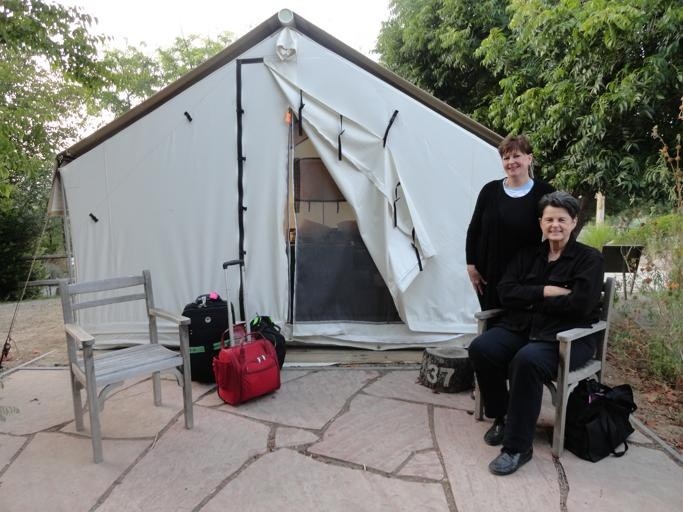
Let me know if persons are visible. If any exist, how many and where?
[465,137,558,327]
[469,192,603,475]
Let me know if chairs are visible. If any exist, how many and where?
[475,276,619,461]
[58,269,196,462]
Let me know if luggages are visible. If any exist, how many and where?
[212,259,282,406]
[179,295,235,385]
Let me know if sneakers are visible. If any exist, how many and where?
[489,446,533,475]
[484,418,503,446]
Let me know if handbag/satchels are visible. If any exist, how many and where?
[563,376,638,463]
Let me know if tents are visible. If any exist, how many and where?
[57,9,505,350]
